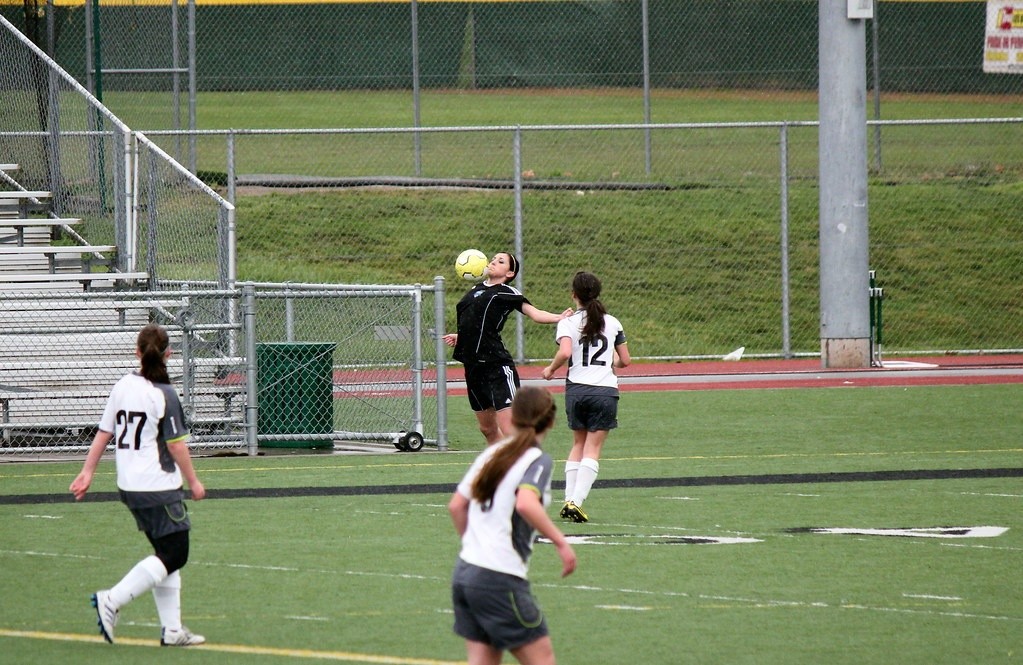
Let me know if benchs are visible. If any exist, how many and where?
[0,163,247,441]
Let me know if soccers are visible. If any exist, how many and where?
[454,248,490,282]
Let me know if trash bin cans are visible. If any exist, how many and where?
[254,340,339,449]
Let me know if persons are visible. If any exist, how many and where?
[542,272,631,523]
[441,252,574,446]
[70,324,205,646]
[450,386,576,665]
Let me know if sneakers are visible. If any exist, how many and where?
[89,591,115,644]
[567,504,588,523]
[560,502,572,518]
[161,627,206,647]
